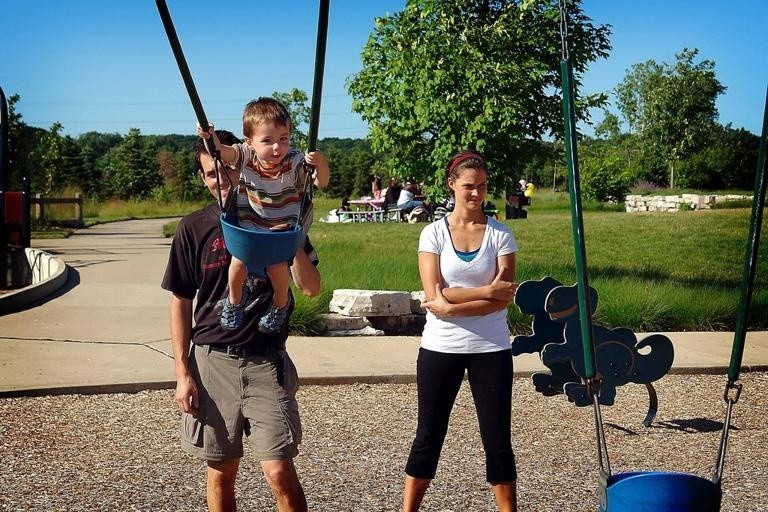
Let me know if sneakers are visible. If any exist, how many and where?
[220,284,251,334]
[258,290,294,336]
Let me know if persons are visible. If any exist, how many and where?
[196,97,330,335]
[160,131,321,512]
[339,175,426,221]
[403,150,519,512]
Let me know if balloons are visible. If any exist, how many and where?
[519,180,534,198]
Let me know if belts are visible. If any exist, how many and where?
[197,342,286,356]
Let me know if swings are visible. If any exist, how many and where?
[560,1,768,510]
[156,0,330,275]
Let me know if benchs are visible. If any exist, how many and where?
[337,193,500,224]
